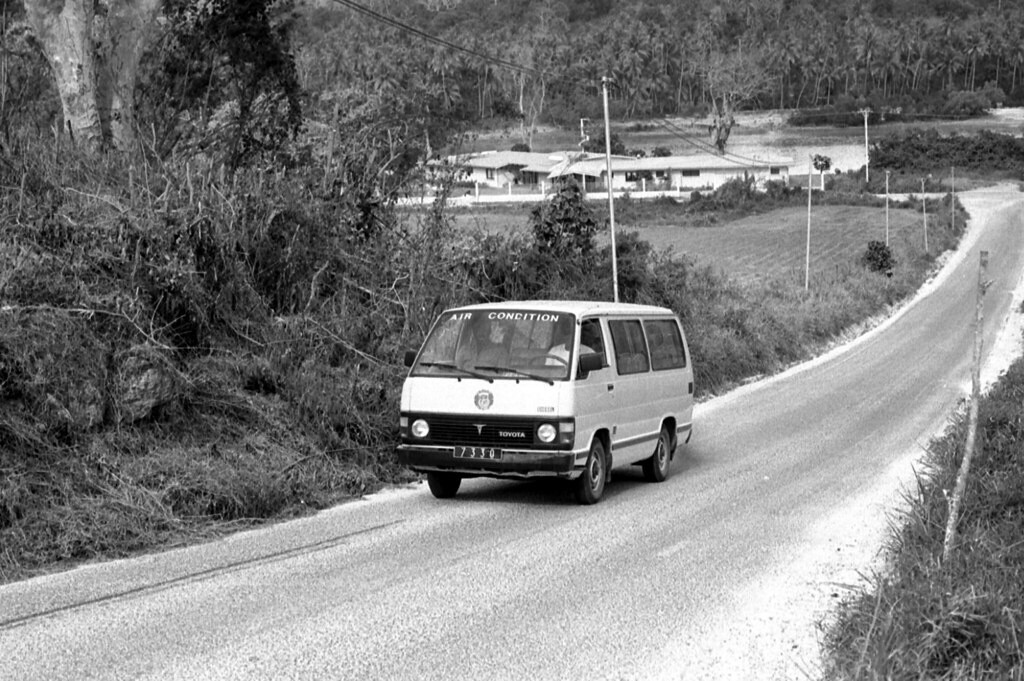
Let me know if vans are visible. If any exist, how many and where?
[396,297,696,505]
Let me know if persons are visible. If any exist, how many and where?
[455,318,507,369]
[545,319,596,366]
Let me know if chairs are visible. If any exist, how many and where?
[476,347,509,373]
[618,354,647,373]
[651,345,682,371]
